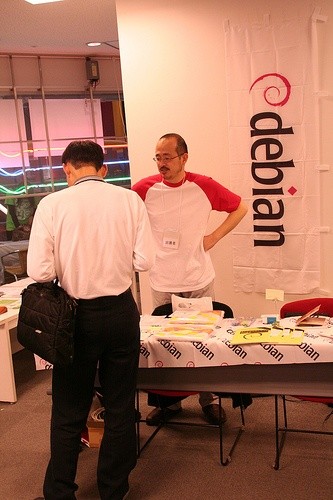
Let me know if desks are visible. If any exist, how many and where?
[35,314,333,398]
[0,277,61,404]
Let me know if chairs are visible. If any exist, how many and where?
[275,297,333,469]
[136,301,246,466]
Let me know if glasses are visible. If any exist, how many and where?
[153,153,183,163]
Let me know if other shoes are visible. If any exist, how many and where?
[109,483,130,500]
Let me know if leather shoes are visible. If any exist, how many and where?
[146,407,182,425]
[202,404,226,425]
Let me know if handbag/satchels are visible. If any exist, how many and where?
[16,281,77,364]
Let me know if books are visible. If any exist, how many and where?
[230,305,326,346]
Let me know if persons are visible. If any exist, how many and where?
[127,133,249,426]
[26,141,155,500]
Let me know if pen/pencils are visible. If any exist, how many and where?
[240,330,268,333]
